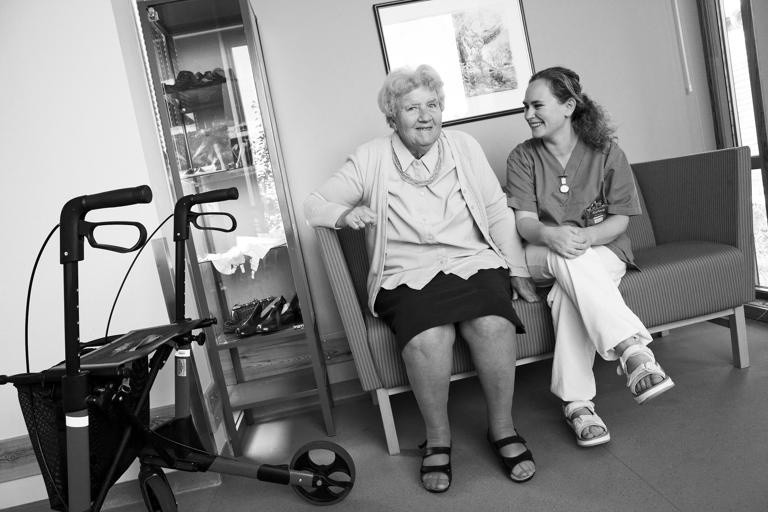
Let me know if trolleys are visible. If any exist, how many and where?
[0,184,356,512]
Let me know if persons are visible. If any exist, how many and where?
[504,63,676,451]
[300,62,541,496]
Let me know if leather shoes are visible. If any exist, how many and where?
[235,293,302,336]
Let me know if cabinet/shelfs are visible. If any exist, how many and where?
[147,1,338,457]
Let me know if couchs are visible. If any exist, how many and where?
[312,142,756,461]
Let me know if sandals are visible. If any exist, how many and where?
[486,424,536,483]
[560,401,611,446]
[417,437,451,492]
[616,344,675,407]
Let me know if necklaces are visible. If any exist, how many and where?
[387,133,446,189]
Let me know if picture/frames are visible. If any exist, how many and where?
[373,0,538,133]
